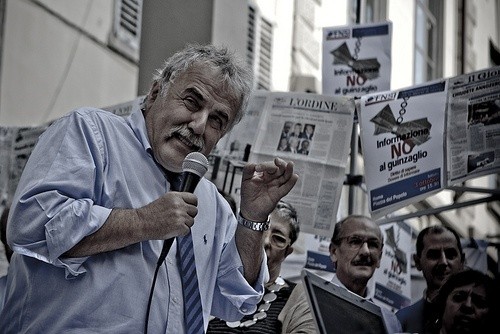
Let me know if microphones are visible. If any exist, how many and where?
[157,152,208,266]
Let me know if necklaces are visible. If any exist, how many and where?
[220,274,284,329]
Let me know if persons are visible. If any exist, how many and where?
[277,122,315,156]
[0,40,299,334]
[208,199,500,334]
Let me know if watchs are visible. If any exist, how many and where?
[236,209,271,232]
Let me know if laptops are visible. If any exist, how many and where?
[301,269,389,334]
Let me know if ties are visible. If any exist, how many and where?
[167,171,205,334]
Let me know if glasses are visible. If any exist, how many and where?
[264,232,290,251]
[339,235,383,250]
